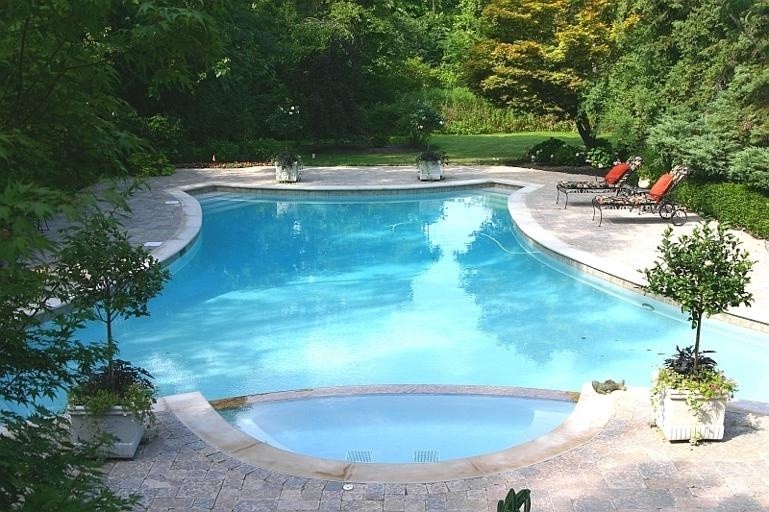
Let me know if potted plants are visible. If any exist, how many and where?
[46,200,174,462]
[644,211,761,445]
[413,148,448,184]
[273,151,304,185]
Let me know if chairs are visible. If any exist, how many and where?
[553,153,690,226]
